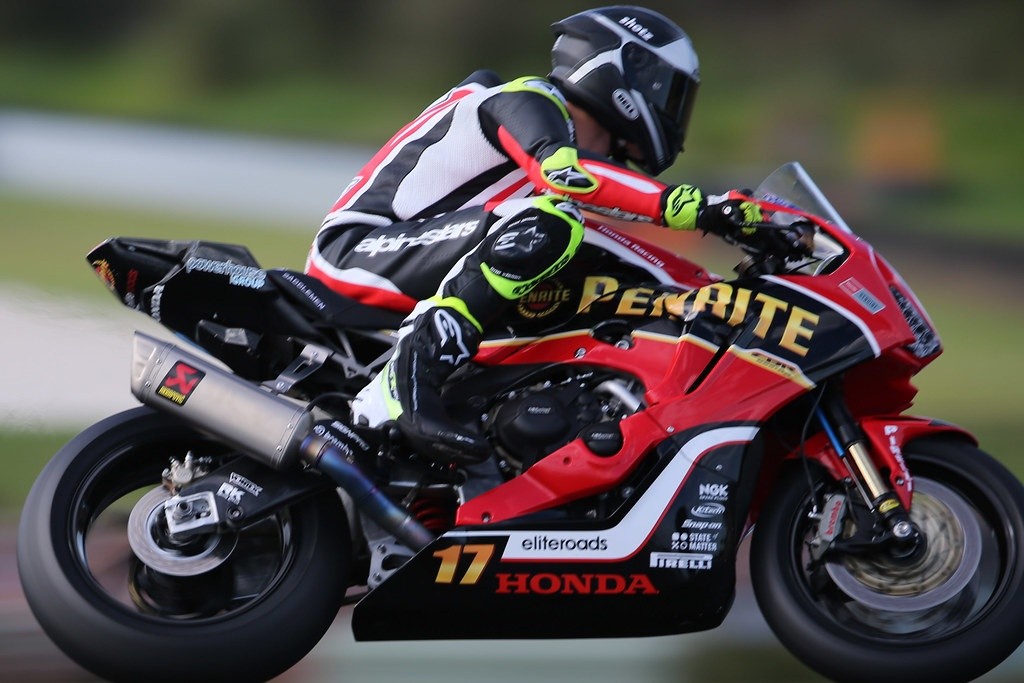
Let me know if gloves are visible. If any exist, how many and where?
[703,189,817,264]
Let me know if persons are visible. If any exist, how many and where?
[303,5,814,466]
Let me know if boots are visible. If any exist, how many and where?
[350,296,494,464]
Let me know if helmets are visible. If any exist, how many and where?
[547,5,700,176]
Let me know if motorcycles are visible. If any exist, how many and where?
[13,160,1024,683]
